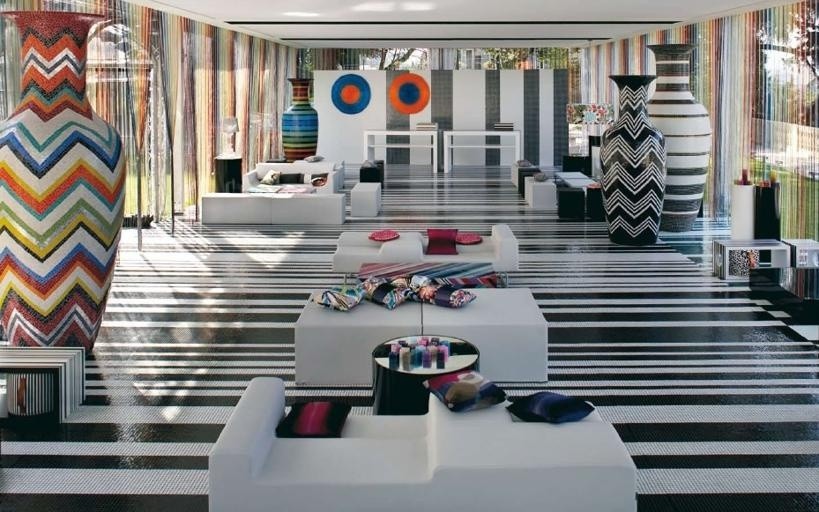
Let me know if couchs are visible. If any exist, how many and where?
[243,162,337,194]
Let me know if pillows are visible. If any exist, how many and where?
[260,170,328,187]
[427,229,458,256]
[422,372,504,412]
[274,400,350,437]
[310,275,477,314]
[507,391,594,426]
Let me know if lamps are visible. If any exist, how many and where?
[222,117,239,157]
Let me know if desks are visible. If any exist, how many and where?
[443,130,522,175]
[363,129,438,175]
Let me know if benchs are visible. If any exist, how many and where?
[360,161,384,189]
[295,286,548,386]
[332,224,519,288]
[511,165,558,212]
[0,347,85,423]
[202,192,346,226]
[208,377,636,512]
[350,183,381,217]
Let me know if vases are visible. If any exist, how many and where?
[282,79,318,162]
[0,11,126,356]
[600,75,668,247]
[646,44,712,232]
